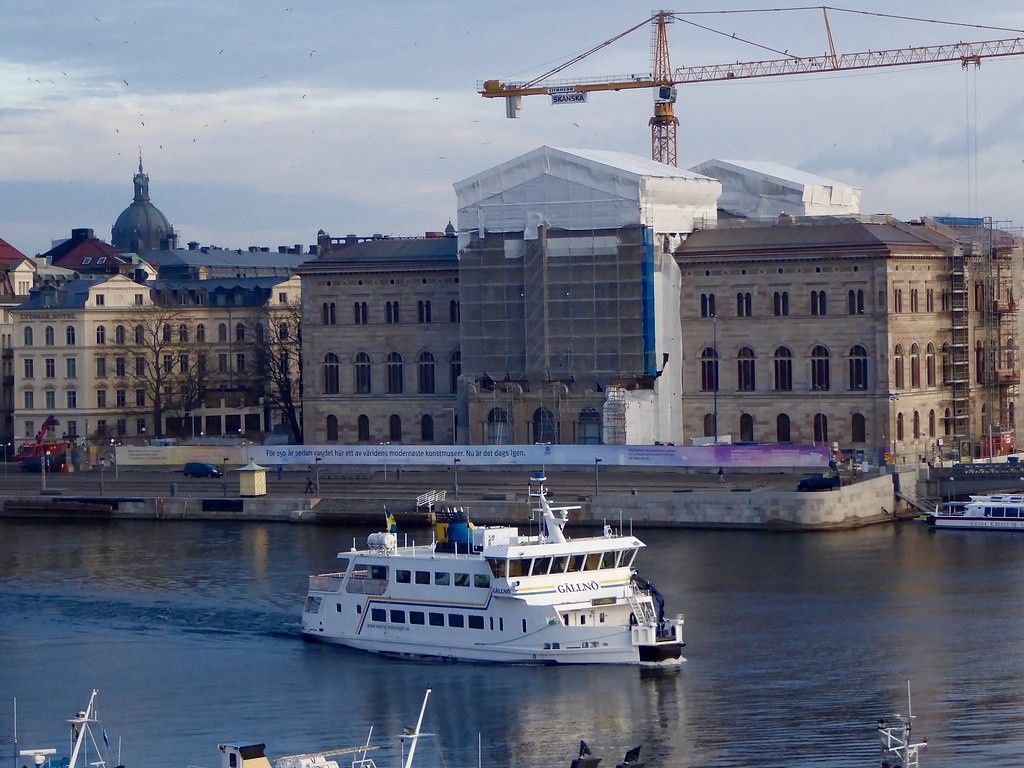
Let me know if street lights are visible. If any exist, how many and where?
[223,456,229,498]
[442,408,456,445]
[315,455,322,496]
[0,442,11,476]
[710,313,719,443]
[535,442,553,478]
[595,456,602,497]
[452,456,461,499]
[99,457,105,497]
[814,384,826,447]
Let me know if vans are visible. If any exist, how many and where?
[183,461,225,479]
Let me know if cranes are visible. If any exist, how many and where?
[473,7,1024,168]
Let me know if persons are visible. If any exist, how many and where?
[304,477,314,494]
[277,464,283,480]
[996,448,1000,456]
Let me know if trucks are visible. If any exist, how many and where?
[17,414,70,473]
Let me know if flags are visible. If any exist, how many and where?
[383,505,398,534]
[72,722,79,742]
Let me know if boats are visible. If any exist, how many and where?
[912,494,1024,529]
[300,471,687,665]
